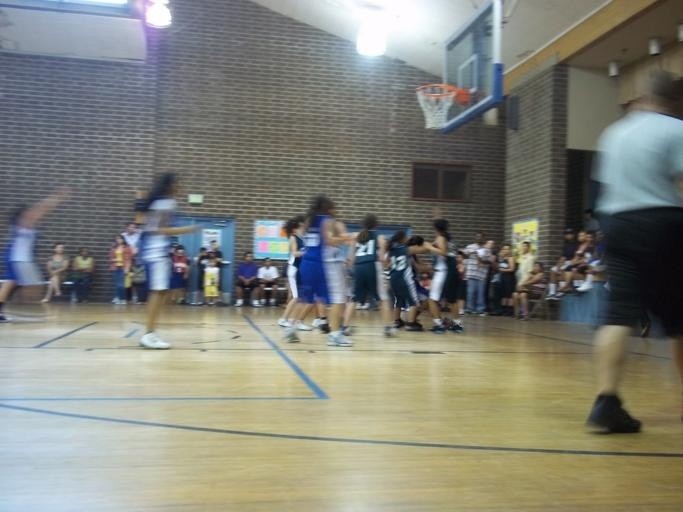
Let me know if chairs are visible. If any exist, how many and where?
[517,278,553,323]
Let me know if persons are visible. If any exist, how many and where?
[584,69,681,431]
[0,171,652,351]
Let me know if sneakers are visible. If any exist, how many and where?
[384,318,463,336]
[234,299,276,307]
[586,396,640,434]
[545,285,594,301]
[285,334,301,342]
[327,336,352,347]
[458,310,529,321]
[113,298,147,305]
[355,301,381,311]
[140,333,170,349]
[278,318,353,336]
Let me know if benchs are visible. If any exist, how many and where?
[11,278,86,303]
[243,283,288,304]
